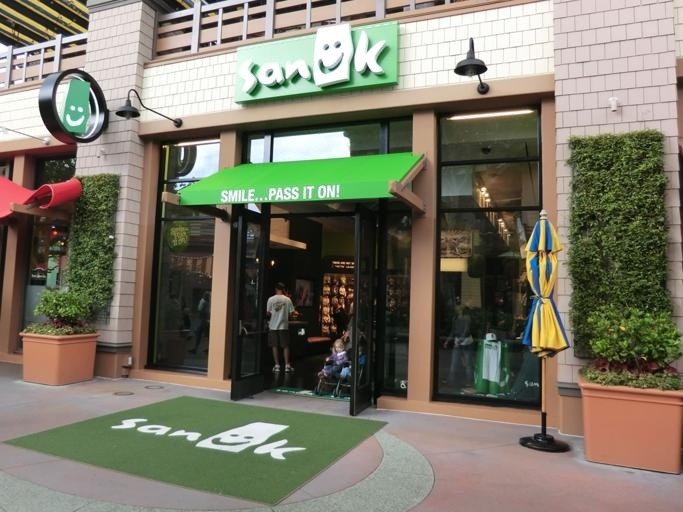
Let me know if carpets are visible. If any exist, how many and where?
[1,393,390,506]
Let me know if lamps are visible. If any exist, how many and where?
[453,36,491,99]
[114,87,184,131]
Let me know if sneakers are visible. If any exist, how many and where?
[272,364,280,372]
[285,365,295,372]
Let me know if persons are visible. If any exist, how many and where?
[317,338,348,379]
[340,317,352,340]
[265,282,298,372]
[329,303,348,337]
[186,273,209,356]
[440,304,474,386]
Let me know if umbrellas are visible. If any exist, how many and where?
[521,209,571,434]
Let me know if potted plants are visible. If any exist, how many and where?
[19,287,102,386]
[156,306,192,365]
[574,302,683,476]
